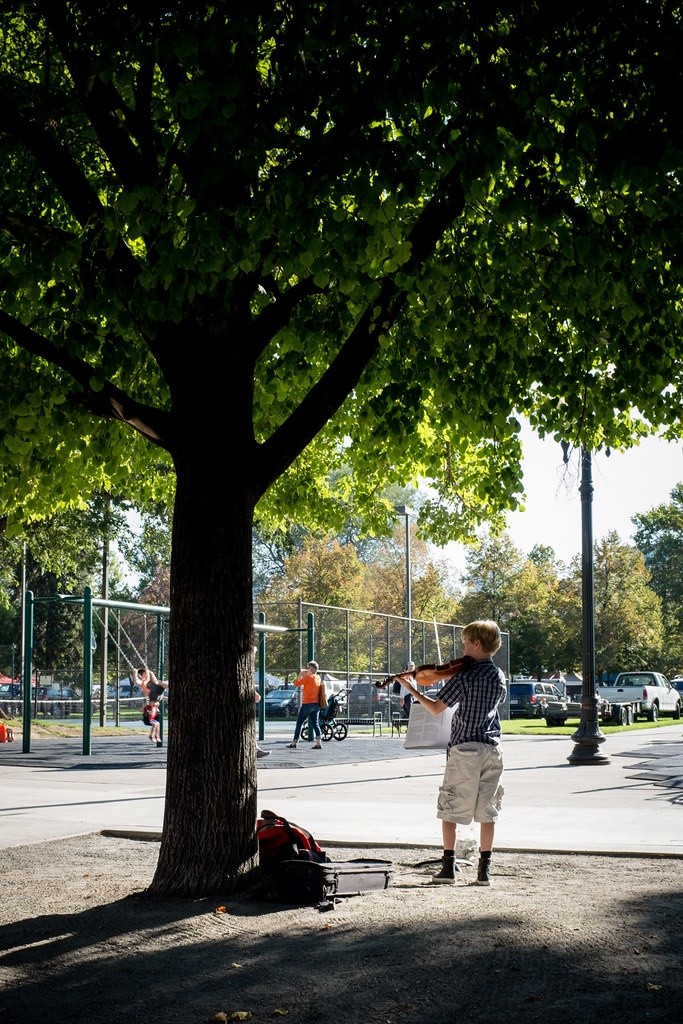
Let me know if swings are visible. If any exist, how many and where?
[92,607,164,701]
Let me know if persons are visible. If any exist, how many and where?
[134,669,169,743]
[286,659,321,750]
[540,697,548,715]
[254,690,269,758]
[399,660,417,716]
[395,620,507,886]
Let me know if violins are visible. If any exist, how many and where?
[375,655,476,691]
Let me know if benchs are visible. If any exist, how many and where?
[333,711,383,738]
[390,712,408,737]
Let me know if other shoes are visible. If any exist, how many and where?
[286,744,296,748]
[257,749,269,758]
[311,744,322,749]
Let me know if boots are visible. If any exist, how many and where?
[476,858,491,886]
[432,856,455,883]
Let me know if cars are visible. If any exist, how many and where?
[256,683,298,717]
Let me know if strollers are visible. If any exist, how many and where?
[300,688,352,742]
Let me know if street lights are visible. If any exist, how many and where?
[391,506,413,661]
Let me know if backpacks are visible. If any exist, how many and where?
[256,809,329,873]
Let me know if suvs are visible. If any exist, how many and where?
[347,681,441,718]
[669,675,683,717]
[507,682,569,719]
[0,676,168,719]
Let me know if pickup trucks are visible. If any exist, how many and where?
[596,671,682,722]
